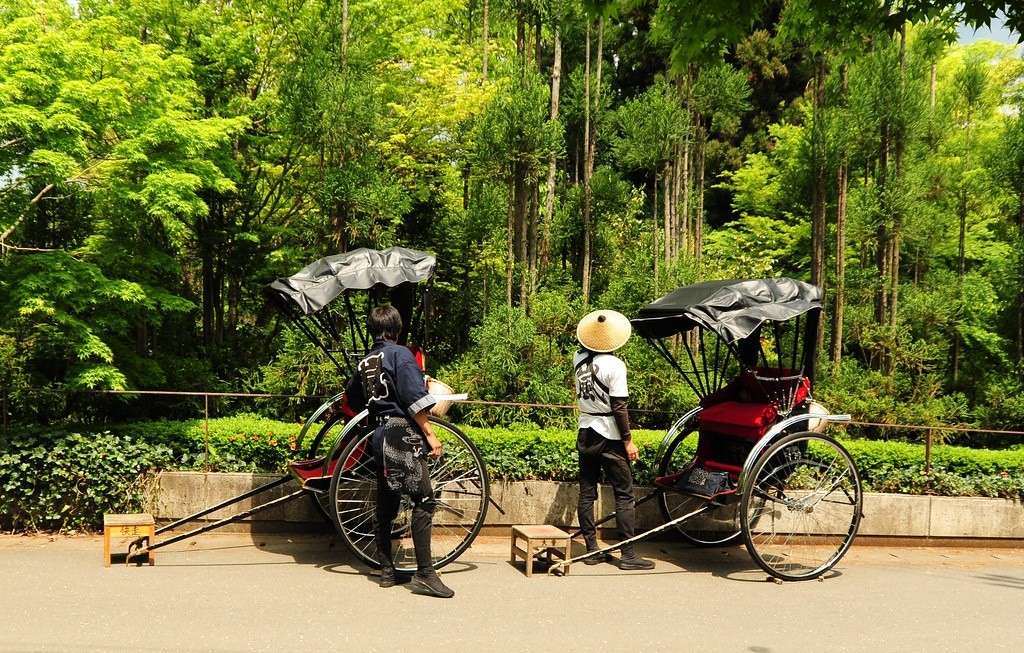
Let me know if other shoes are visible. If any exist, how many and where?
[412,571,454,598]
[380,567,412,587]
[585,550,613,565]
[620,557,656,569]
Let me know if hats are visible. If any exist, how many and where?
[576,310,631,352]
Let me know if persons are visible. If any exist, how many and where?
[572,310,656,569]
[345,304,454,599]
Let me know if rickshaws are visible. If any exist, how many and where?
[544,276,865,582]
[125,245,493,581]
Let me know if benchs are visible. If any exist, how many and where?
[284,344,425,496]
[693,364,811,443]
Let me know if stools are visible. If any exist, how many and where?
[102,513,156,567]
[510,524,572,579]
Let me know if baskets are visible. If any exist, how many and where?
[423,377,456,416]
